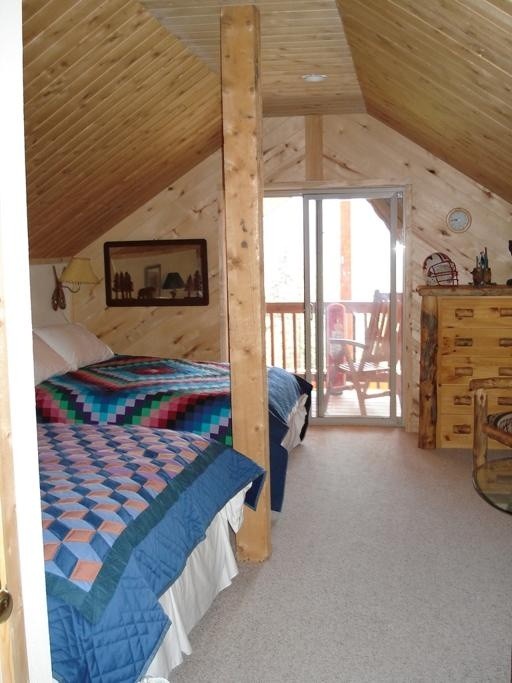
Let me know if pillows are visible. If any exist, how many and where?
[33,320,112,386]
[34,334,68,386]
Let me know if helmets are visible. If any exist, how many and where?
[424,253,458,286]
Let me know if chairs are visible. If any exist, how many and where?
[469,377,512,514]
[322,289,404,417]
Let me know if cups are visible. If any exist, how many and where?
[472,267,491,286]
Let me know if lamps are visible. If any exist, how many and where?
[51,257,97,311]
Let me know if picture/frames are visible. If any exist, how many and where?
[104,239,209,306]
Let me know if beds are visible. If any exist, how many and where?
[36,354,313,510]
[36,423,267,683]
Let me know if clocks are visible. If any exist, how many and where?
[446,208,472,234]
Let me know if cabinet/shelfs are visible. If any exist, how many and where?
[418,285,512,449]
